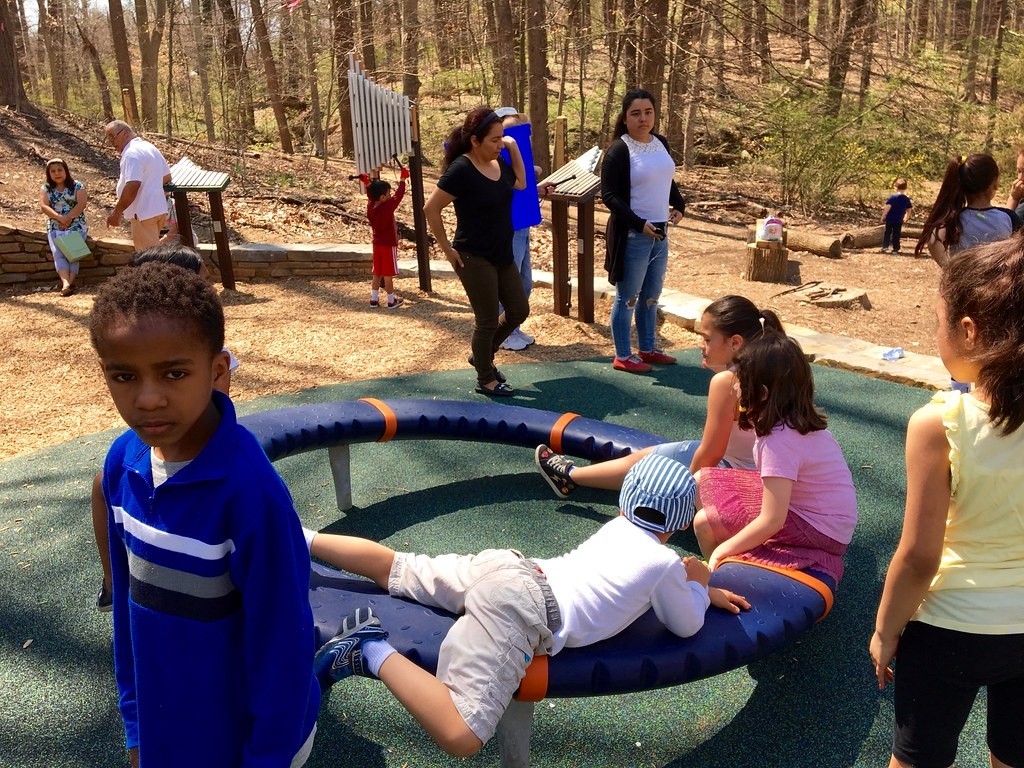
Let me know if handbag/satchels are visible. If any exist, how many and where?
[53,227,91,263]
[756,214,774,243]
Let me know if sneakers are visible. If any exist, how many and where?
[499,335,529,350]
[370,297,379,307]
[510,327,535,344]
[314,606,389,692]
[388,298,403,309]
[613,356,652,371]
[536,445,576,499]
[637,349,677,363]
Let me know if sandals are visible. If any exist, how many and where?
[476,381,515,395]
[469,355,505,381]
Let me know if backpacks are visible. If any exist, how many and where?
[761,215,782,241]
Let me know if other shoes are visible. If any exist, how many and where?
[894,250,898,254]
[62,286,70,295]
[881,248,886,253]
[95,578,113,611]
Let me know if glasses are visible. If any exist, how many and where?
[110,129,124,141]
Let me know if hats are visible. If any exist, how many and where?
[618,453,697,534]
[493,107,528,123]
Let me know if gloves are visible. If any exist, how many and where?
[401,168,410,178]
[359,173,369,185]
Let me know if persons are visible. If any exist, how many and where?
[492,108,557,350]
[90,261,321,768]
[423,105,530,396]
[105,120,199,253]
[300,452,752,756]
[40,157,88,296]
[600,88,685,373]
[359,166,410,310]
[535,294,786,499]
[880,179,913,254]
[91,239,239,613]
[869,227,1024,768]
[692,328,858,573]
[916,149,1024,394]
